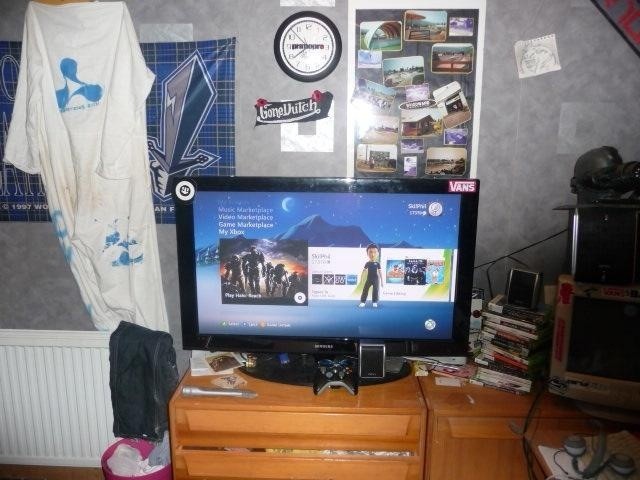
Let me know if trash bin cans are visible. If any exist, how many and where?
[101,437,172,480]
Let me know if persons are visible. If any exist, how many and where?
[358,243,383,308]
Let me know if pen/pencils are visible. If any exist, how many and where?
[466,393,474,404]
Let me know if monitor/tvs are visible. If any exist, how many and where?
[172,176,479,386]
[548,275,640,423]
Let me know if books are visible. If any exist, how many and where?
[469,295,554,396]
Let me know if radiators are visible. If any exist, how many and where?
[0,327,123,468]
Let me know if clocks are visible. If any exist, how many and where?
[275,9,342,82]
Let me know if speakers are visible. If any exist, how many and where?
[568,210,639,283]
[505,267,543,311]
[358,339,385,379]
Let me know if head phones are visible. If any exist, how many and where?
[562,434,635,477]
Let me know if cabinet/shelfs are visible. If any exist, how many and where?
[413,360,590,480]
[167,355,428,479]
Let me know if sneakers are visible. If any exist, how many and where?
[359,303,365,307]
[373,303,377,307]
